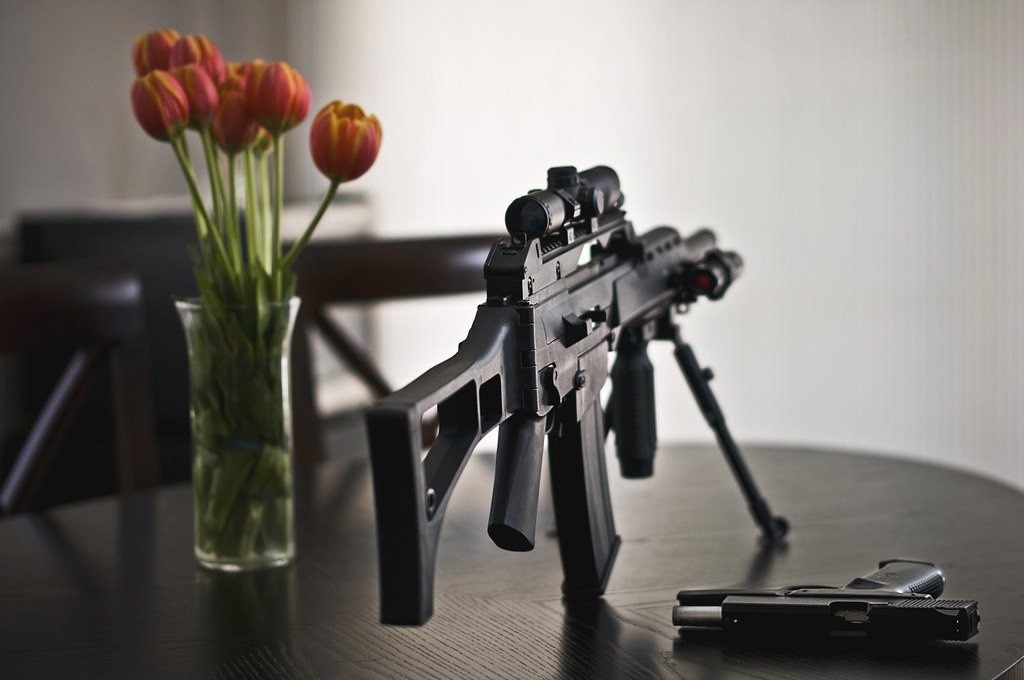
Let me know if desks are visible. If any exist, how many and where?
[0,437,1024,680]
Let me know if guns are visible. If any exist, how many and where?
[674,560,979,644]
[367,163,790,626]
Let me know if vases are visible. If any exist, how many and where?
[170,298,305,571]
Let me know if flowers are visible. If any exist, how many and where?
[128,25,382,561]
